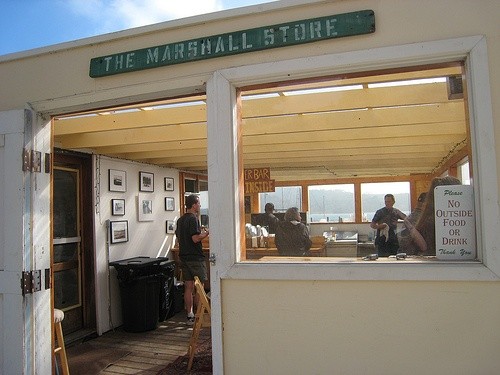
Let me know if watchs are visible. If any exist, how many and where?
[205,230,209,234]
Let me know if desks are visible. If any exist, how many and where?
[170,236,326,288]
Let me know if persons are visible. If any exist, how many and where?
[402,177,463,254]
[370,194,407,256]
[175,194,209,326]
[274,207,312,256]
[254,203,280,234]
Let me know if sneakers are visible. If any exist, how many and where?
[187,313,195,326]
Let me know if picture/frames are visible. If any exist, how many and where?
[140,171,154,191]
[165,177,175,190]
[112,199,125,215]
[109,169,127,192]
[165,197,175,211]
[139,193,156,221]
[167,220,176,233]
[111,220,128,244]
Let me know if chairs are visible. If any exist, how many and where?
[186,276,211,372]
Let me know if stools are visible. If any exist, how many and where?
[54,308,69,375]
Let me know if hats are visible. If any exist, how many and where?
[417,191,430,202]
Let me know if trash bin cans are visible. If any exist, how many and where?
[148,258,177,322]
[108,255,168,331]
